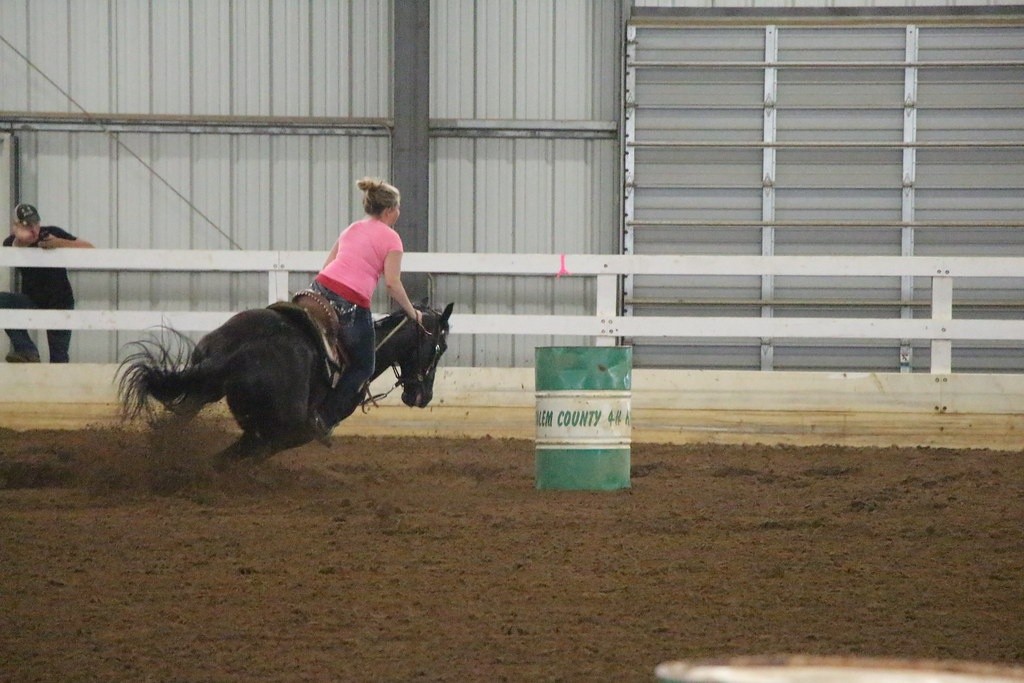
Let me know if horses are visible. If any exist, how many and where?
[108,287,456,484]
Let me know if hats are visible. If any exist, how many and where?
[13,204,40,226]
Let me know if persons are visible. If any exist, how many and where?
[0,204,95,363]
[309,176,423,448]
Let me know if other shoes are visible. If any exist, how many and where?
[306,409,332,448]
[6,351,41,363]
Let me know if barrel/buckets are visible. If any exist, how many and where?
[532,343,633,489]
